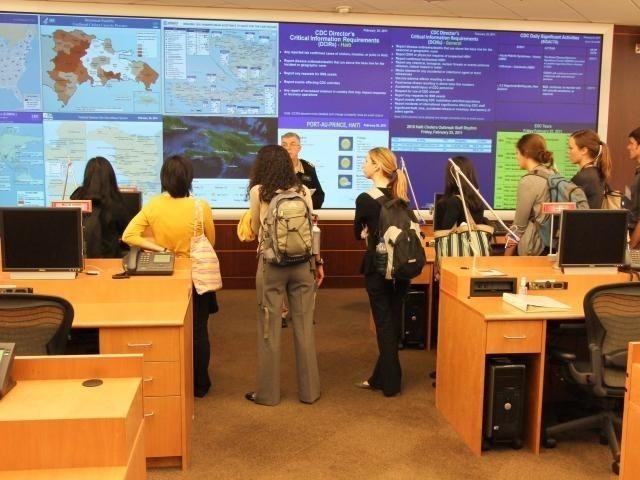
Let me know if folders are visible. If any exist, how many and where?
[501,291,573,314]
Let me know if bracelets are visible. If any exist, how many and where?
[160,247,170,253]
[121,153,223,398]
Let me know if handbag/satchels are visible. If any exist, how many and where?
[435,192,495,274]
[602,178,622,210]
[190,200,227,295]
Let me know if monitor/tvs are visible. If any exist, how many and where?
[555,208,629,275]
[0,206,85,280]
[121,191,141,222]
[432,191,448,232]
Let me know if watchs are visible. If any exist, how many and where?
[313,259,325,267]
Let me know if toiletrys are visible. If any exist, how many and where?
[519,278,527,294]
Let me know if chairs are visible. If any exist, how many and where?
[0,291,75,355]
[545,281,640,474]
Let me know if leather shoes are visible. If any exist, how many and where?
[246,391,266,403]
[353,380,374,389]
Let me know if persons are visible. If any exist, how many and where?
[568,129,614,210]
[269,132,325,328]
[427,155,495,386]
[69,156,137,259]
[352,147,411,397]
[503,134,566,256]
[236,145,325,406]
[622,126,640,252]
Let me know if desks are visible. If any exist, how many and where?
[0,256,195,470]
[367,224,439,351]
[0,352,147,480]
[433,256,640,459]
[617,341,640,480]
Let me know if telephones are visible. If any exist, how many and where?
[111,243,176,280]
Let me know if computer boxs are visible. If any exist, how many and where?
[402,289,428,345]
[483,359,528,443]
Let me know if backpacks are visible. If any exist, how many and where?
[256,183,316,266]
[521,165,591,254]
[361,188,427,284]
